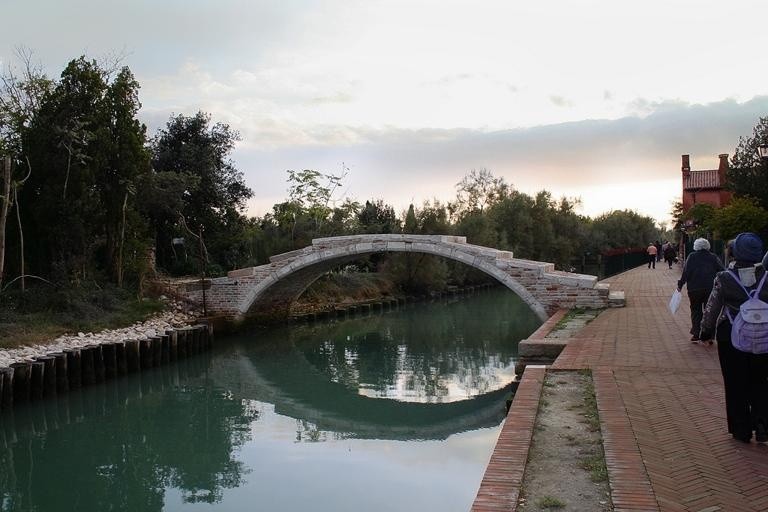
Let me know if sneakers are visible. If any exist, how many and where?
[691,336,699,343]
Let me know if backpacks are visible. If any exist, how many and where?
[725,269,768,354]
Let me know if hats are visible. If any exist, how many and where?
[732,233,763,262]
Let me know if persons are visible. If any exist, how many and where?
[677,238,727,341]
[700,232,768,443]
[646,240,678,269]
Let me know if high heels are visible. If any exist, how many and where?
[733,422,768,443]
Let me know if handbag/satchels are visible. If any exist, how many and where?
[669,289,682,315]
